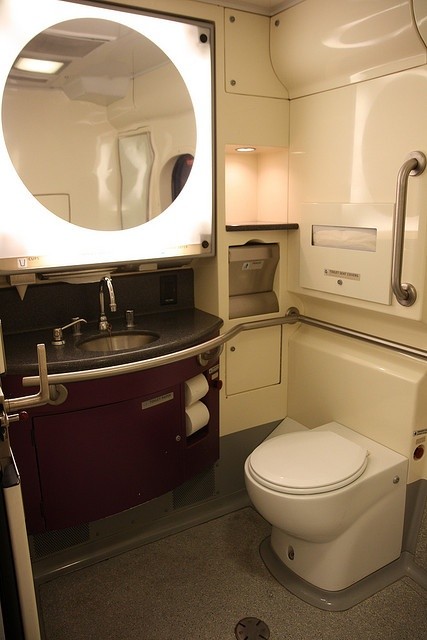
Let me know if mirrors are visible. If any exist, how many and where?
[0,0,217,278]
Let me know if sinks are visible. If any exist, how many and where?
[74,331,160,351]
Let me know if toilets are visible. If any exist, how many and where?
[244,421,409,592]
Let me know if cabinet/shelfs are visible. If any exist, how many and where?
[27,386,194,534]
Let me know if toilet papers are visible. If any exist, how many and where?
[184,373,210,407]
[185,401,210,437]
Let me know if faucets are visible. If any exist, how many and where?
[99,276,117,331]
[51,319,88,346]
